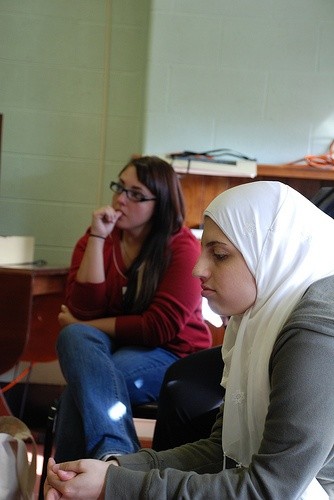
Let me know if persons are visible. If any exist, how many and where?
[40,155,212,500]
[40,179,334,500]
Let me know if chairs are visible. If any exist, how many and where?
[38,237,227,500]
[150,344,229,452]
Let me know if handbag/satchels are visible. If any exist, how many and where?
[0,415,38,500]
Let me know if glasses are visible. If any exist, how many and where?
[109,181,156,202]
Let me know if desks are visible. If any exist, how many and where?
[0,265,70,376]
[132,154,334,231]
